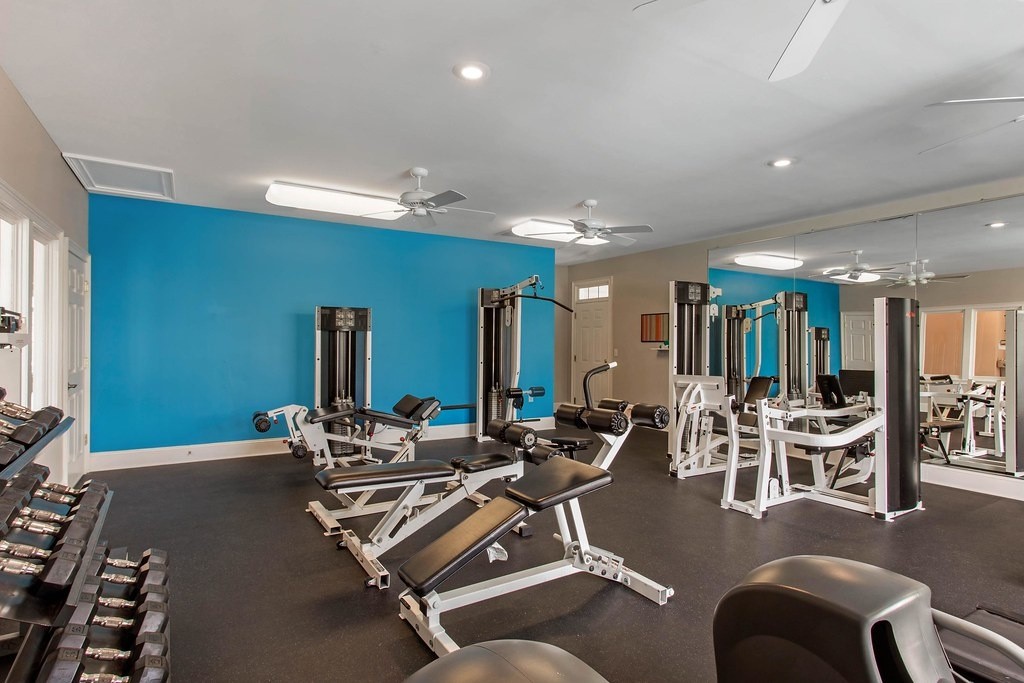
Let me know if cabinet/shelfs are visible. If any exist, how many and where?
[1,332,173,683]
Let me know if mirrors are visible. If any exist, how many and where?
[705,194,1024,482]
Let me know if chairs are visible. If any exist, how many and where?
[816,373,866,427]
[745,376,775,404]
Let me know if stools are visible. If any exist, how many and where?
[920,420,967,464]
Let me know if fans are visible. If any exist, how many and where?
[881,262,957,291]
[524,197,654,250]
[359,167,467,226]
[807,249,903,280]
[905,258,970,285]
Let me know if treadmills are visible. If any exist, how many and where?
[940,602,1024,683]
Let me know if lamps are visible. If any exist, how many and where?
[734,252,804,272]
[823,267,881,283]
[511,217,612,246]
[265,180,411,221]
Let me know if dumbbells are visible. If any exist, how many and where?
[0,387,171,683]
